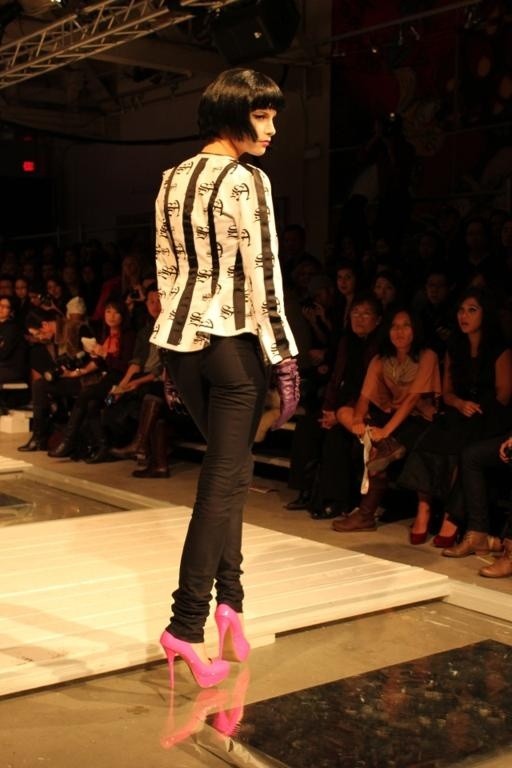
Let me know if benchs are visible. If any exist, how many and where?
[180,418,297,470]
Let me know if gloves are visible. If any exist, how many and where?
[271,359,301,432]
[163,368,189,415]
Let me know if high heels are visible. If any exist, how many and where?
[161,629,228,689]
[216,604,250,662]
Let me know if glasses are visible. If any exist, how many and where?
[352,311,371,319]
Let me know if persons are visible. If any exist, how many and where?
[1,236,196,480]
[244,168,512,577]
[147,68,301,691]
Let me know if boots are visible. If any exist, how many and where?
[333,475,383,530]
[18,405,111,462]
[365,430,405,470]
[111,395,168,475]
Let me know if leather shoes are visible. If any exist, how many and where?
[310,503,332,519]
[409,512,512,577]
[288,488,311,510]
